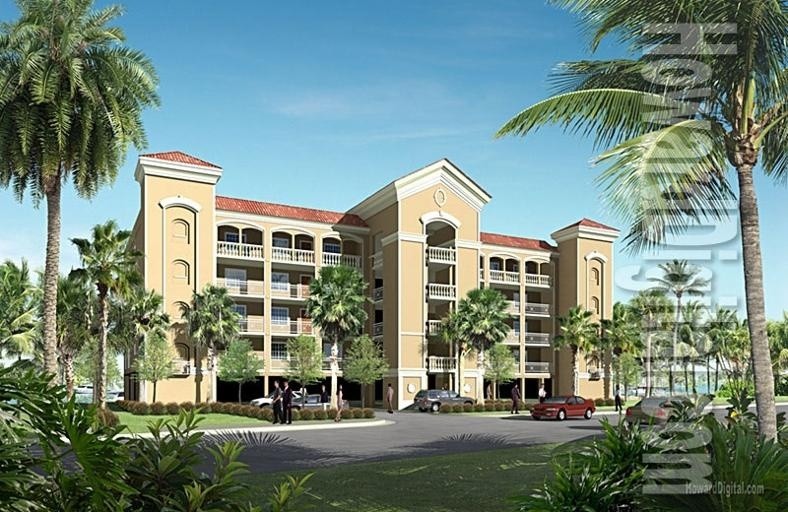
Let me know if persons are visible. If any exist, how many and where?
[614,385,623,412]
[538,383,547,403]
[272,380,282,424]
[280,381,292,424]
[334,384,344,422]
[321,385,328,403]
[511,384,521,414]
[487,384,493,400]
[386,383,394,413]
